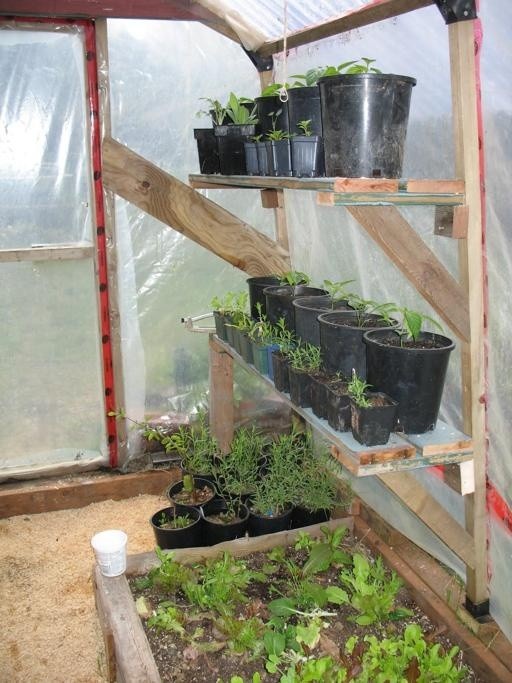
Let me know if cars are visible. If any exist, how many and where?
[88,528,129,578]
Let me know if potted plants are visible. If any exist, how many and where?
[192,56,417,180]
[207,265,455,447]
[149,402,355,549]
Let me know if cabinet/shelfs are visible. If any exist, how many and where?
[189,0,492,624]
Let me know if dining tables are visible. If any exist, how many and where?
[192,56,417,180]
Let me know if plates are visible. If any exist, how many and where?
[189,0,492,624]
